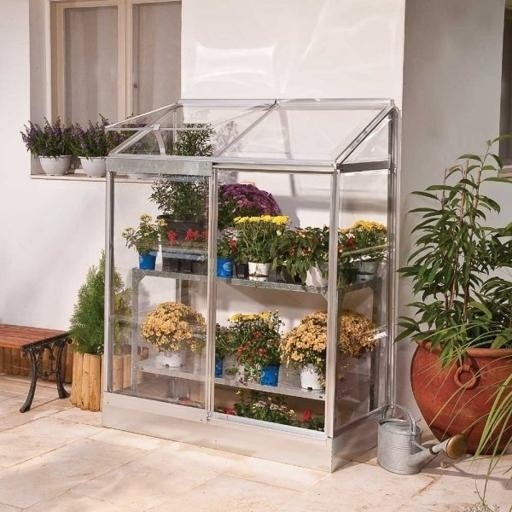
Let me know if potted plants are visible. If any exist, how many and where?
[67,265,139,412]
[398,138,512,459]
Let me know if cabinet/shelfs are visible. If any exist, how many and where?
[102,98,399,474]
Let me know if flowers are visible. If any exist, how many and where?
[123,118,388,432]
[20,116,155,160]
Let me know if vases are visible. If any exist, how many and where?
[35,155,72,176]
[77,155,106,176]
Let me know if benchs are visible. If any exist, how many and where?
[1,324,70,415]
